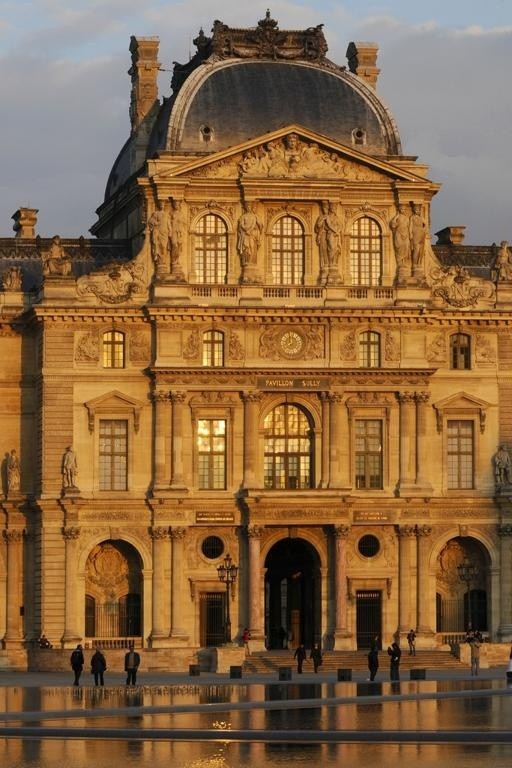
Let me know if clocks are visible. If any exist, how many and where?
[277,327,307,359]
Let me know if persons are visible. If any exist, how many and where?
[392,626,402,648]
[368,646,379,681]
[495,443,510,485]
[390,205,413,277]
[251,205,263,248]
[474,630,482,641]
[309,642,323,674]
[468,638,481,676]
[90,648,107,686]
[496,241,512,280]
[506,647,512,678]
[69,644,85,686]
[407,629,416,656]
[294,644,306,674]
[148,200,173,265]
[125,645,140,686]
[40,635,53,648]
[238,202,256,265]
[1,633,8,649]
[61,446,78,486]
[388,642,401,680]
[201,134,376,182]
[467,629,474,641]
[409,205,428,267]
[7,449,21,491]
[169,199,185,264]
[314,203,342,270]
[243,628,251,656]
[43,235,72,275]
[371,634,379,651]
[285,626,294,654]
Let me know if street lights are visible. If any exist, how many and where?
[217,554,238,643]
[457,557,478,630]
